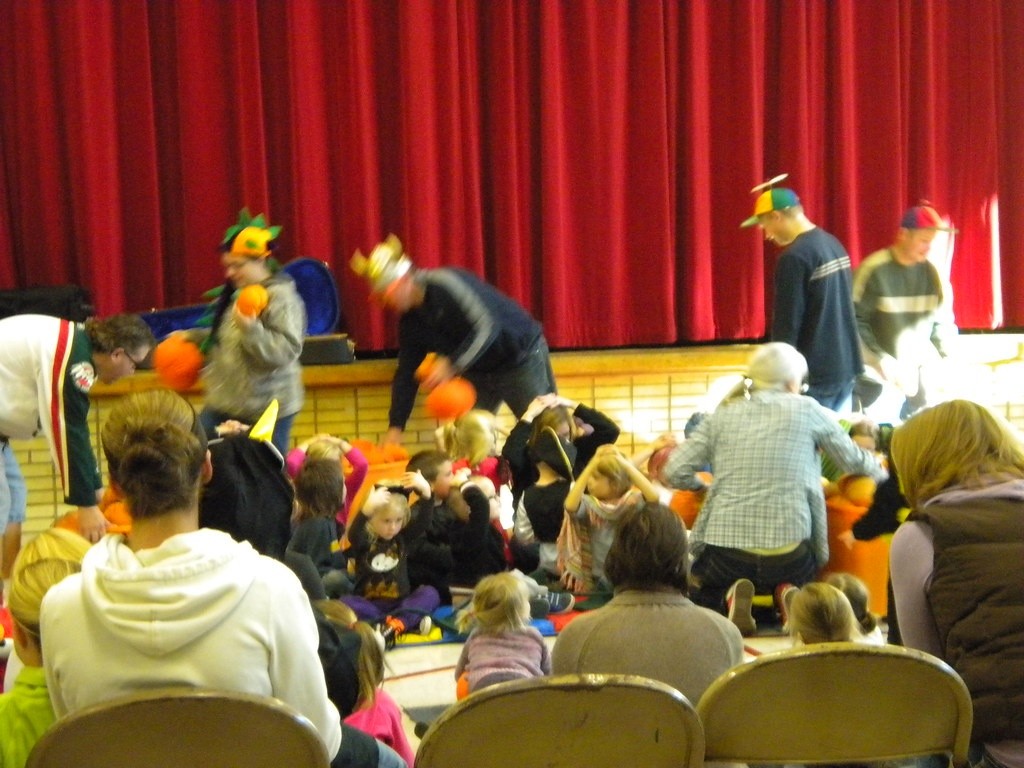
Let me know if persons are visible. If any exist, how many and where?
[368,243,558,450]
[850,204,962,417]
[664,342,891,633]
[0,418,415,768]
[192,215,308,457]
[38,388,342,764]
[0,312,156,609]
[402,393,910,696]
[552,503,745,709]
[741,186,863,412]
[889,399,1024,768]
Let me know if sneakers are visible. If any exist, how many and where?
[374,623,395,652]
[411,616,431,634]
[726,578,756,637]
[538,592,575,614]
[773,582,800,635]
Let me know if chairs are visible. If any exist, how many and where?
[414,670,706,768]
[695,641,975,768]
[24,686,331,768]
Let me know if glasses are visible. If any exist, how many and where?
[123,347,144,370]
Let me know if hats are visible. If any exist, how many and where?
[220,207,282,258]
[349,232,412,293]
[741,187,800,226]
[900,206,959,232]
